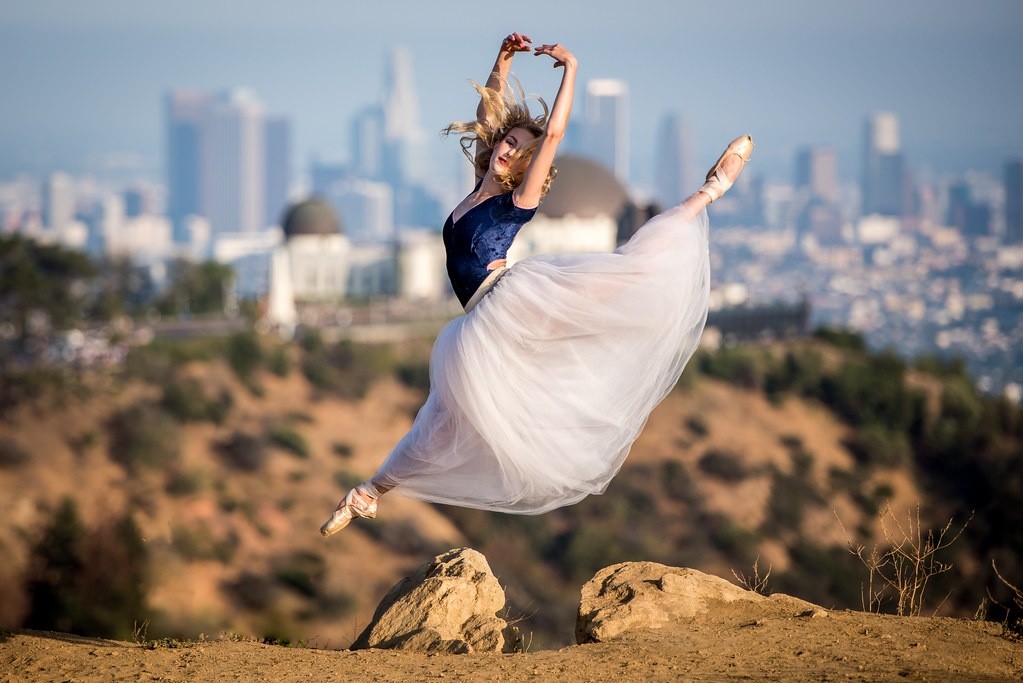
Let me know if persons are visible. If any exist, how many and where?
[320,32,754,536]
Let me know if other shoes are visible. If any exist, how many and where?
[706,134,754,185]
[320,488,377,536]
